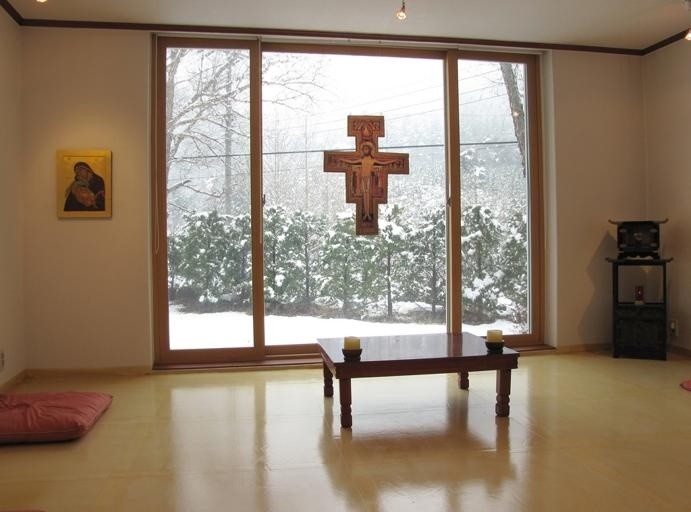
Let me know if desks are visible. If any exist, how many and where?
[317,331,520,428]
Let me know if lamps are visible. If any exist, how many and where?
[397,0,406,19]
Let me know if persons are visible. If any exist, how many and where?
[335,142,400,223]
[62,161,105,211]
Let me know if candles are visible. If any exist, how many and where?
[344,334,360,350]
[487,330,502,342]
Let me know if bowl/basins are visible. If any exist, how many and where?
[342,348,362,360]
[485,341,504,353]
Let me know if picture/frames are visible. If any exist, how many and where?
[55,148,113,218]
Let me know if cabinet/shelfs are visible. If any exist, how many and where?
[604,254,674,361]
